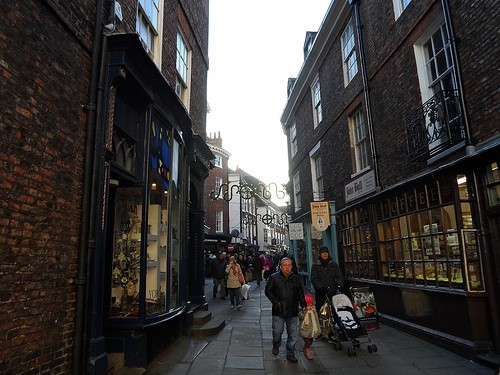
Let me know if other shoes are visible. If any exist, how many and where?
[272,346,279,355]
[221,297,226,300]
[236,304,242,310]
[231,305,234,309]
[304,348,314,360]
[287,354,298,363]
[213,294,216,297]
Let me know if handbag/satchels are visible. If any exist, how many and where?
[241,284,251,300]
[245,270,253,283]
[238,271,244,282]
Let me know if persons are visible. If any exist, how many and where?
[225,256,245,310]
[265,257,307,363]
[298,293,321,359]
[211,251,228,300]
[207,250,298,295]
[312,247,344,340]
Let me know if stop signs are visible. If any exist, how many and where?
[227,244,234,251]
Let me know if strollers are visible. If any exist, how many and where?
[324,285,377,357]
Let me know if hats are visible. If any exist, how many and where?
[229,256,235,261]
[318,246,330,253]
[304,293,314,304]
